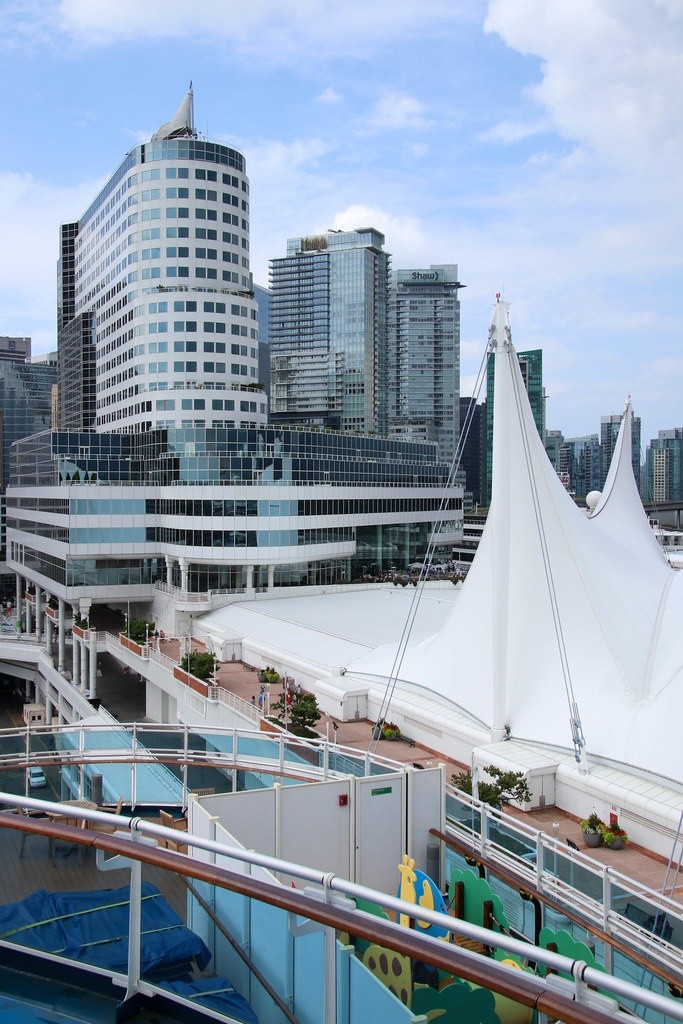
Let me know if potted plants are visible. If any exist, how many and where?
[24,585,37,603]
[449,765,537,864]
[258,666,279,684]
[260,685,328,766]
[173,649,220,697]
[72,612,96,640]
[118,616,156,656]
[45,599,60,619]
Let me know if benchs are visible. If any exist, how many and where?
[615,903,673,943]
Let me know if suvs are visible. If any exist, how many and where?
[28,766,47,788]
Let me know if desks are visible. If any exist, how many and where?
[45,800,97,857]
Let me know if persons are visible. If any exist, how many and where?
[155,630,159,641]
[296,684,303,705]
[122,666,131,676]
[258,692,265,711]
[30,696,35,704]
[251,696,255,707]
[160,630,165,643]
[49,734,56,751]
[96,661,104,681]
[7,607,11,616]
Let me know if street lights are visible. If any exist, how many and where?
[552,822,560,902]
[146,623,149,646]
[325,712,330,742]
[213,656,217,687]
[266,683,271,716]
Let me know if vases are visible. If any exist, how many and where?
[372,729,383,740]
[386,733,397,741]
[583,832,602,847]
[606,836,623,849]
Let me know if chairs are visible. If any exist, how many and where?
[191,788,216,796]
[159,809,188,853]
[18,798,123,868]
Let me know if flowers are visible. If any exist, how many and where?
[579,812,628,847]
[373,718,400,737]
